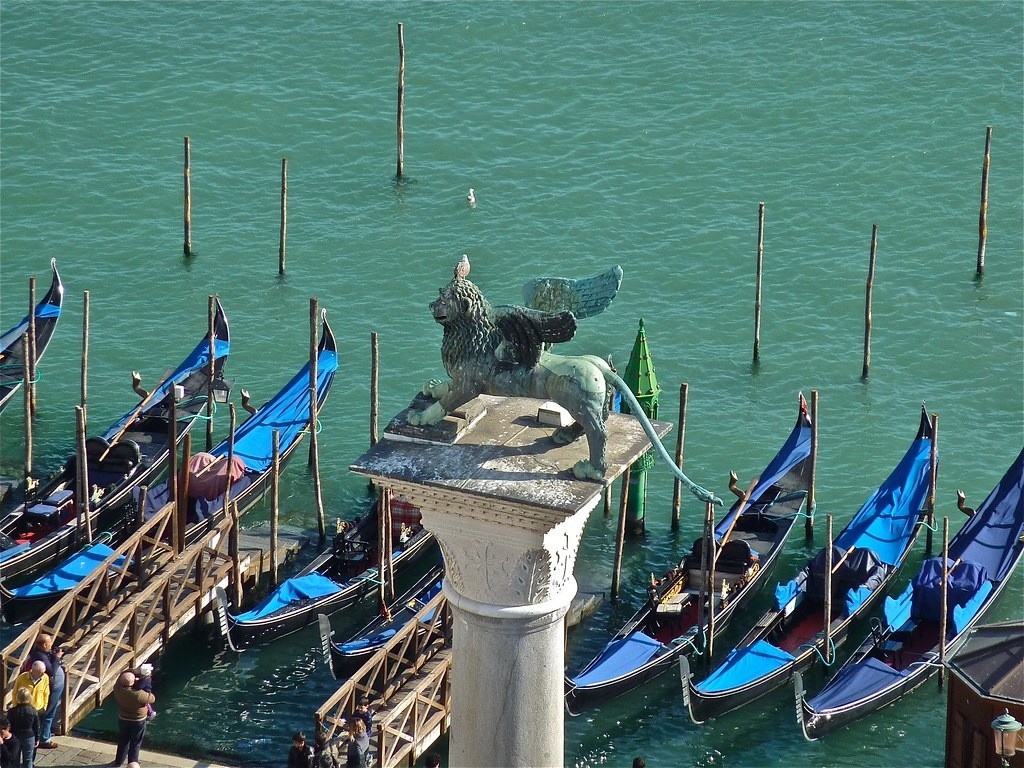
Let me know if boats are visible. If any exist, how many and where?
[317,554,451,685]
[563,389,809,716]
[1,296,232,591]
[0,307,340,627]
[678,397,941,727]
[214,491,434,654]
[0,257,65,416]
[790,445,1024,743]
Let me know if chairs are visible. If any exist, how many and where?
[124,500,157,538]
[647,585,682,636]
[332,531,368,580]
[24,475,58,534]
[869,617,904,668]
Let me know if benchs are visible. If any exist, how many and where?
[679,536,752,594]
[895,612,923,648]
[345,524,381,560]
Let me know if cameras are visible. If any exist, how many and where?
[54,646,70,656]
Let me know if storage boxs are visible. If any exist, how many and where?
[537,400,571,427]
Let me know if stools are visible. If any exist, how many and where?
[44,490,73,511]
[666,592,693,618]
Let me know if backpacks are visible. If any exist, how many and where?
[18,657,33,675]
[329,744,340,768]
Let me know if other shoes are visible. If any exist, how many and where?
[147,711,156,720]
[38,740,58,748]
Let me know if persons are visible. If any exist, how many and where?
[340,718,370,768]
[132,664,156,721]
[424,753,440,768]
[0,718,19,768]
[345,696,373,767]
[127,762,140,768]
[30,634,65,748]
[113,668,155,767]
[7,688,40,768]
[288,731,338,768]
[12,661,50,768]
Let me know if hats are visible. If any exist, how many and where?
[141,664,153,675]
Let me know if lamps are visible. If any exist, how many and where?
[990,707,1024,768]
[209,370,236,404]
